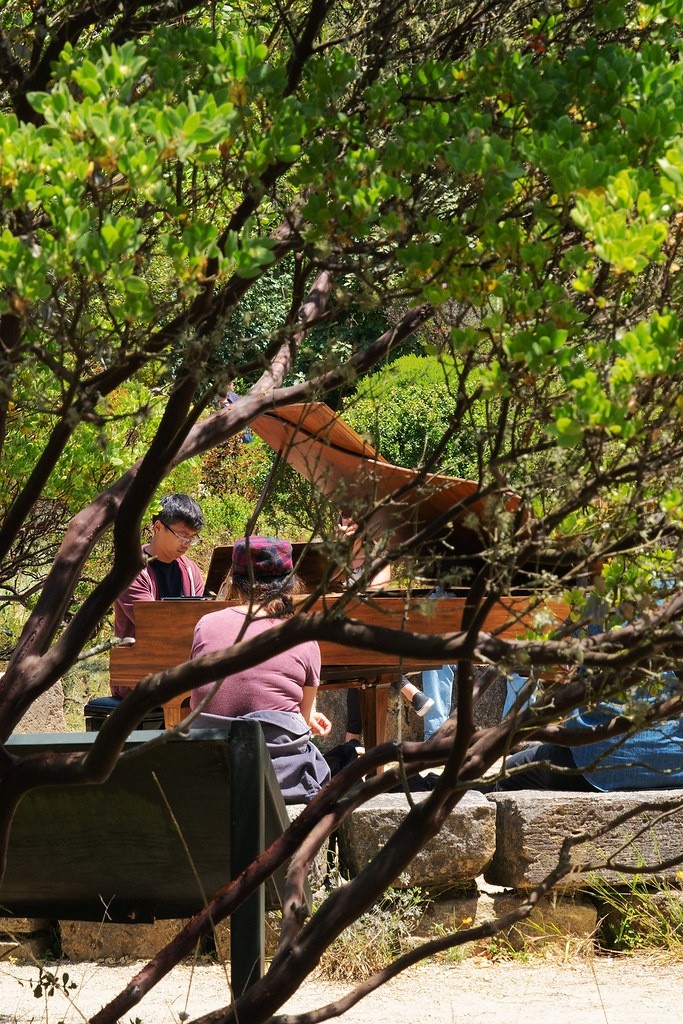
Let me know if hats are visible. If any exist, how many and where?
[232,536,293,576]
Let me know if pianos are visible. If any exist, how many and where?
[108,400,570,752]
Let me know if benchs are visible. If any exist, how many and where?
[1,718,313,1004]
[85,696,191,730]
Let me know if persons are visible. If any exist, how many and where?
[190,535,332,803]
[109,494,204,703]
[336,500,683,790]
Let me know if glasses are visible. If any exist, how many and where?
[161,521,203,547]
